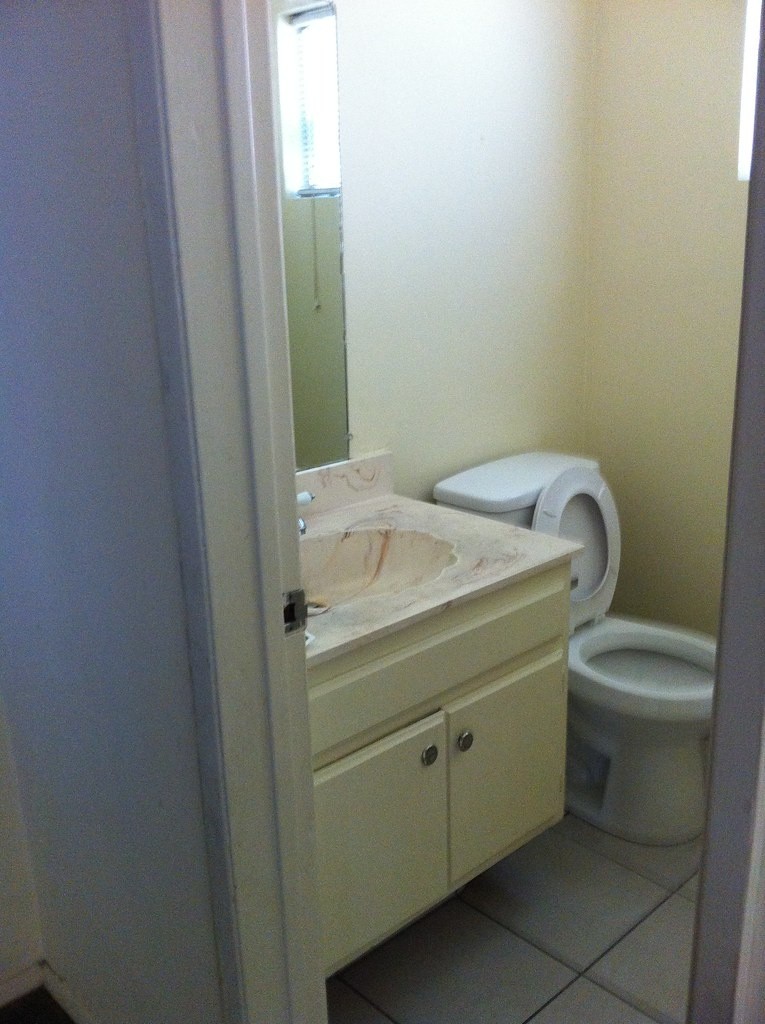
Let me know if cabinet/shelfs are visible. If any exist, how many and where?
[306,559,571,983]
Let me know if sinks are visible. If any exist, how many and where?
[298,527,460,617]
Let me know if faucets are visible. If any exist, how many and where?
[296,490,315,536]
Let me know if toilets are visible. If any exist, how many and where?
[431,450,716,847]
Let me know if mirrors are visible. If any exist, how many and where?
[278,0,352,474]
